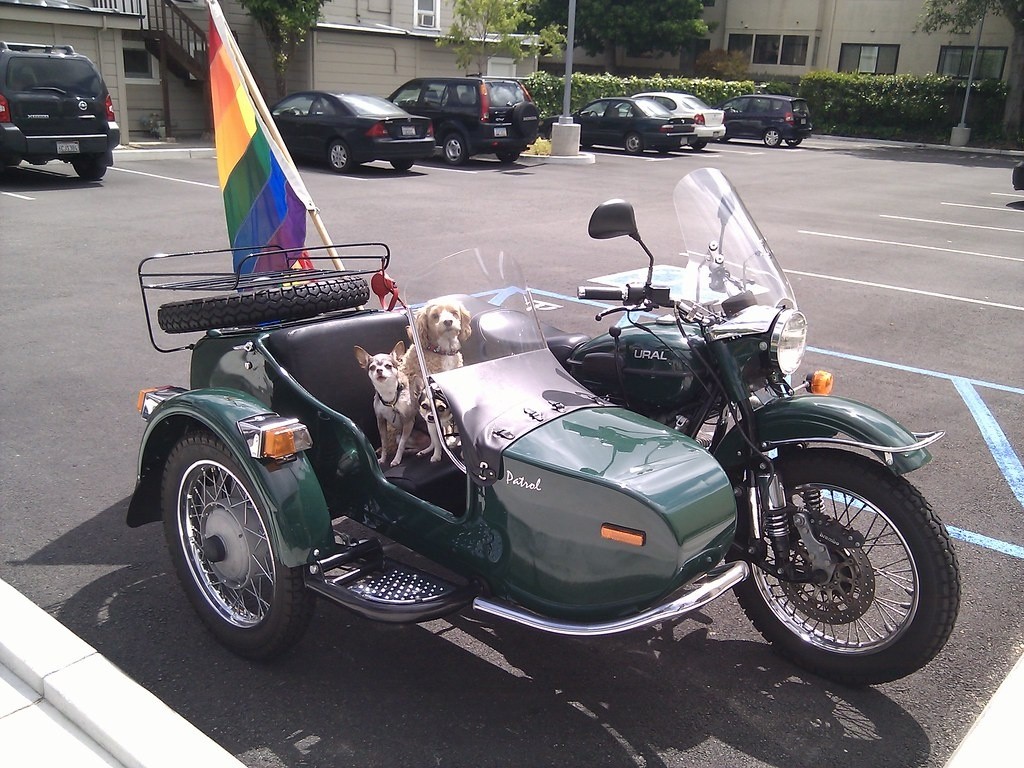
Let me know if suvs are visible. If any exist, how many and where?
[381,75,541,166]
[0,39,120,183]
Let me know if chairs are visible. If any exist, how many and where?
[263,305,465,510]
[423,89,439,102]
[459,92,473,105]
[324,103,335,114]
[608,107,619,117]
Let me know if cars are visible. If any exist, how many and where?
[537,96,699,154]
[597,91,727,150]
[267,89,437,172]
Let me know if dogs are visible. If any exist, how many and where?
[352,295,470,468]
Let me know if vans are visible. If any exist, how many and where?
[711,93,812,148]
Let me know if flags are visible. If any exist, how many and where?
[207,1,308,298]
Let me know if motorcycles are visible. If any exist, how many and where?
[122,167,963,689]
